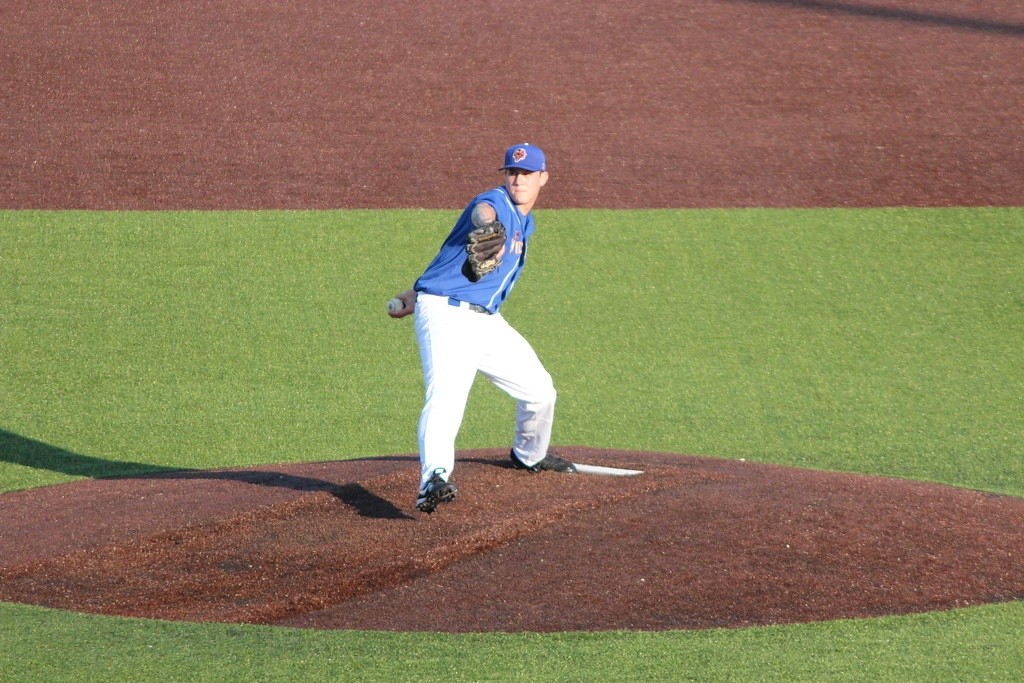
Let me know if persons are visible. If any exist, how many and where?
[388,142,576,514]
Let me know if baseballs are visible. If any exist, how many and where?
[387,298,403,312]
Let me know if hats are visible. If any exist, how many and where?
[498,143,545,171]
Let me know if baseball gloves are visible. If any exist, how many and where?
[465,220,507,282]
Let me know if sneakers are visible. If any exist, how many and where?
[511,448,577,474]
[415,468,458,516]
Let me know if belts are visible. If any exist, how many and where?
[415,293,493,315]
